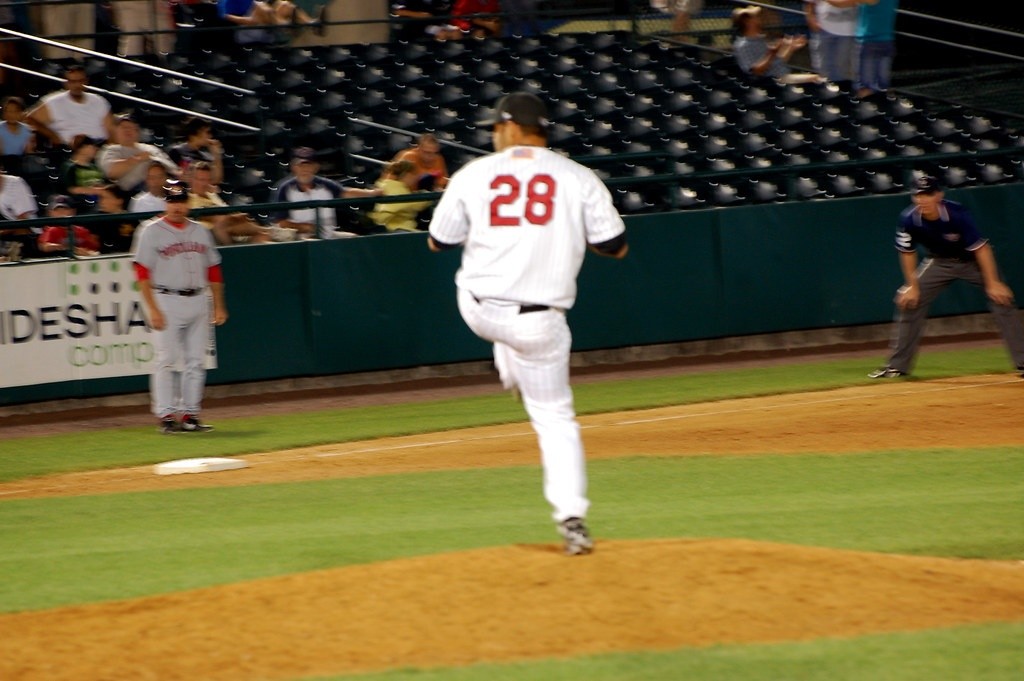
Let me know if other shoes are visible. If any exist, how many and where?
[557,520,593,554]
[158,417,186,434]
[868,366,905,380]
[180,415,213,432]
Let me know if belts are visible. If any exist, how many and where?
[472,295,551,314]
[151,285,204,297]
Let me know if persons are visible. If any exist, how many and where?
[650,0,706,43]
[867,177,1024,378]
[732,0,898,97]
[0,65,449,256]
[428,93,628,554]
[167,0,329,53]
[132,186,228,434]
[388,0,501,42]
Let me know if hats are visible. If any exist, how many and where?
[289,146,321,165]
[911,177,941,196]
[162,178,189,203]
[474,93,551,128]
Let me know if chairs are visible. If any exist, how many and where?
[1,37,1023,263]
